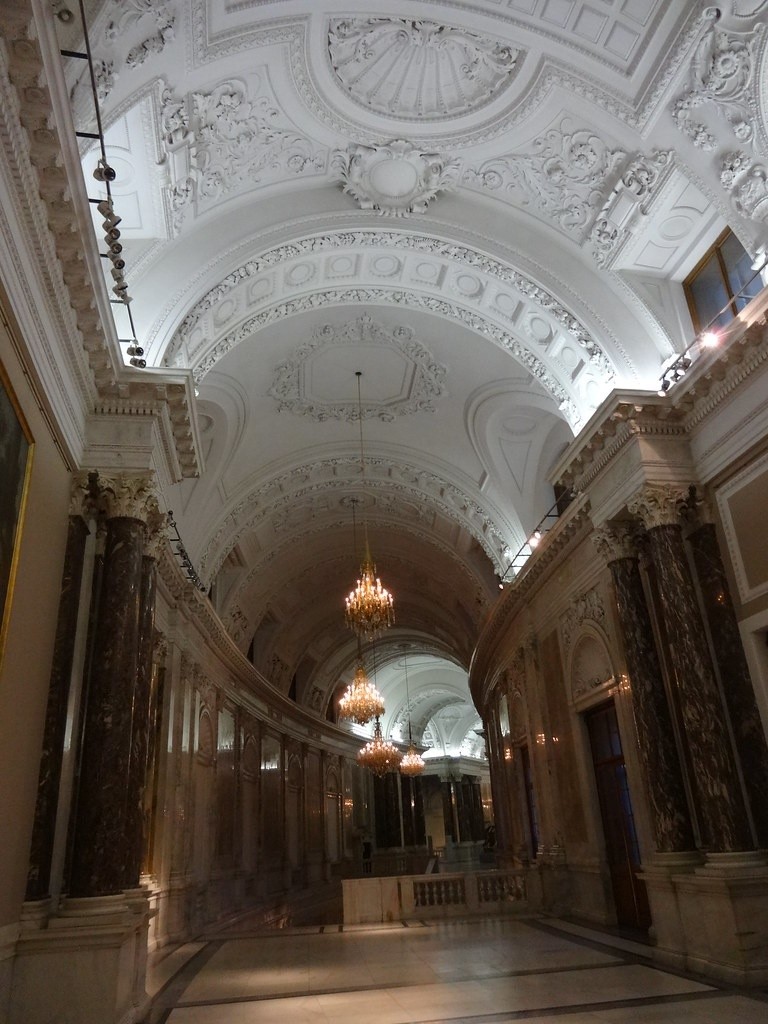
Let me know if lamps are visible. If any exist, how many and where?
[337,499,386,726]
[398,642,426,780]
[356,641,402,779]
[345,370,395,644]
